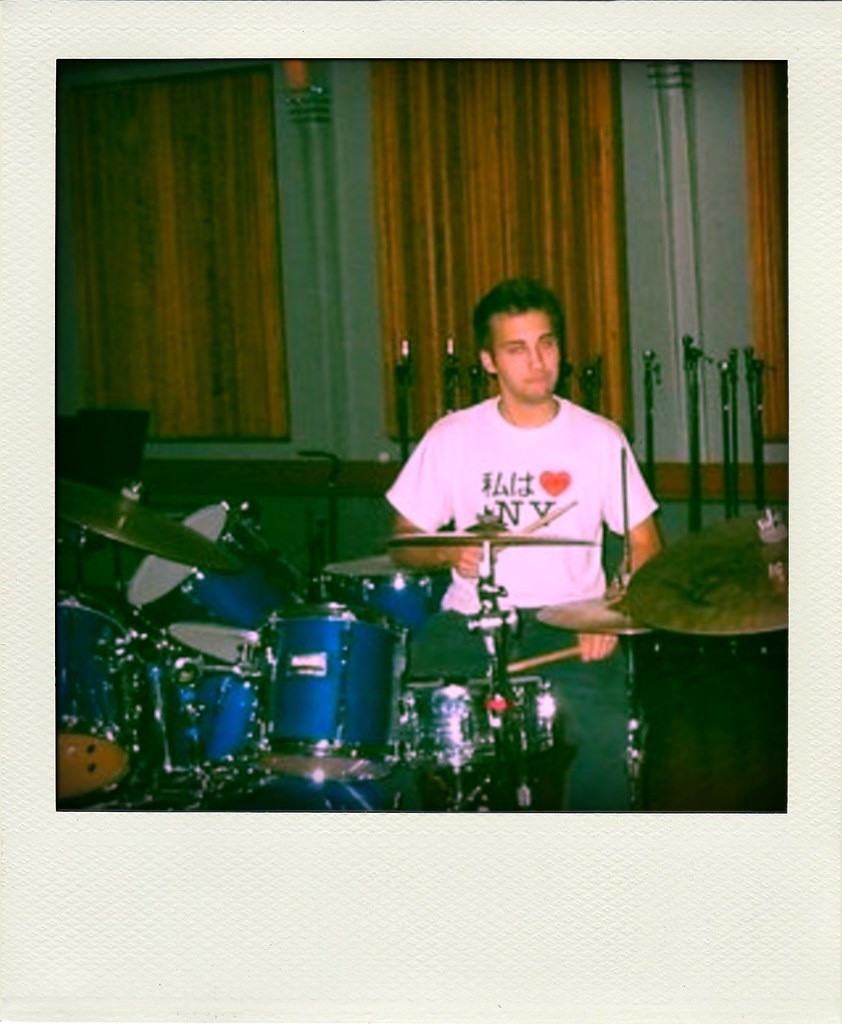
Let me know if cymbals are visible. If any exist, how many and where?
[382,519,593,545]
[55,479,235,569]
[537,590,648,635]
[626,502,787,634]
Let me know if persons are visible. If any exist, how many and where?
[380,271,669,814]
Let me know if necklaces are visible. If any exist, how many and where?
[501,399,558,427]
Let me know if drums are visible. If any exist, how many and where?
[163,620,266,762]
[56,591,138,803]
[129,501,308,635]
[402,676,552,768]
[322,553,453,638]
[253,750,396,812]
[271,602,409,754]
